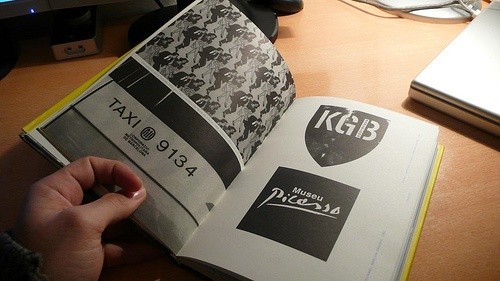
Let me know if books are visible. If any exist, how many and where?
[20,0,444,281]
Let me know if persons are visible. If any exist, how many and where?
[0,156,146,281]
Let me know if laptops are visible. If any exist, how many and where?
[409,0,500,137]
